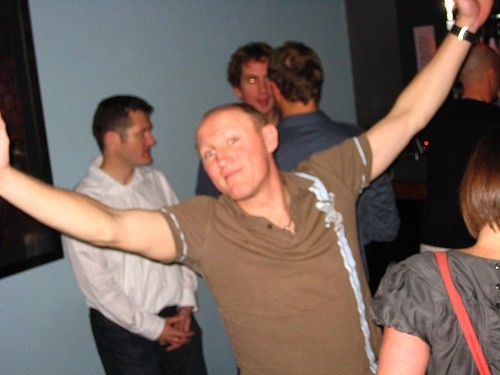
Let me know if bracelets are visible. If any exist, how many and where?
[450,25,477,43]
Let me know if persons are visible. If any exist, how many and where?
[0,0,500,375]
